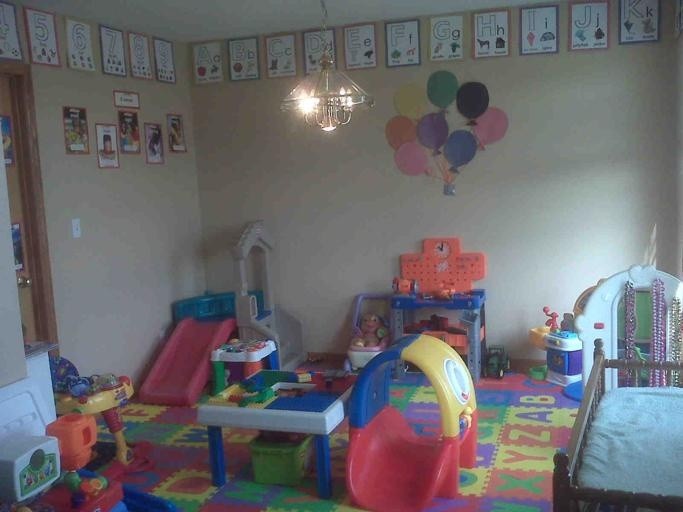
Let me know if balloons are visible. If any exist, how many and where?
[385,70,509,176]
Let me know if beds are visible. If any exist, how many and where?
[552,339,682,511]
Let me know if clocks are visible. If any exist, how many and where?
[436,241,452,260]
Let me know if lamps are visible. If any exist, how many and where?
[278,1,376,133]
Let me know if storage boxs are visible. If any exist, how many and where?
[251,432,316,486]
